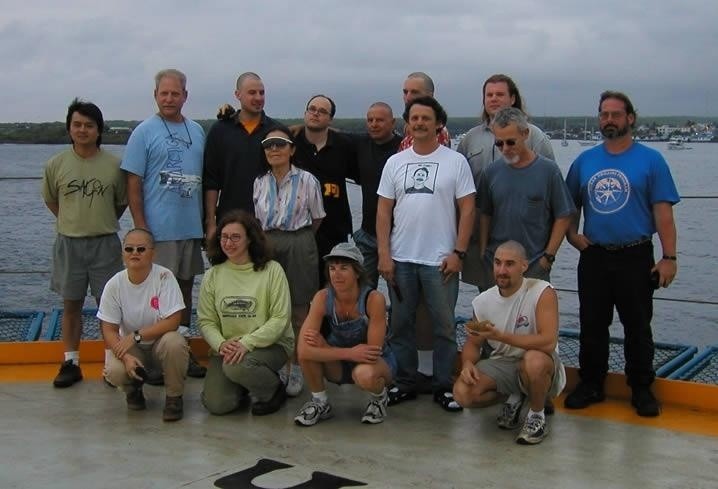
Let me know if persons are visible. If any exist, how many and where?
[474,108,576,415]
[456,72,555,292]
[97,229,191,422]
[217,92,361,335]
[196,208,296,417]
[252,124,327,397]
[287,102,404,289]
[41,98,127,389]
[562,90,681,417]
[452,240,567,445]
[292,241,398,427]
[397,71,452,152]
[120,69,208,386]
[374,97,478,413]
[202,72,297,266]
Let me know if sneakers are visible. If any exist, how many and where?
[631,387,659,416]
[144,366,165,385]
[294,399,336,427]
[516,413,549,445]
[564,384,606,409]
[127,388,146,410]
[286,373,305,397]
[187,355,207,378]
[495,395,526,430]
[53,359,82,388]
[252,380,288,416]
[361,393,391,424]
[163,396,184,421]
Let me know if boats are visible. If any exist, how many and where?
[667,141,692,151]
[578,140,597,146]
[454,134,465,145]
[560,142,569,146]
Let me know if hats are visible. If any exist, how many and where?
[323,243,364,264]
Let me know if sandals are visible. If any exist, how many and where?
[433,388,463,412]
[388,383,417,405]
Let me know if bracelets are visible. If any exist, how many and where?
[661,255,679,261]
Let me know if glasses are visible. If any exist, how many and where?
[494,139,516,148]
[260,135,293,148]
[122,246,154,252]
[598,110,624,119]
[308,105,333,116]
[218,231,241,243]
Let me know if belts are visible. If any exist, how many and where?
[604,236,650,251]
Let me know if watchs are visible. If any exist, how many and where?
[132,327,141,348]
[543,252,557,265]
[453,249,469,263]
